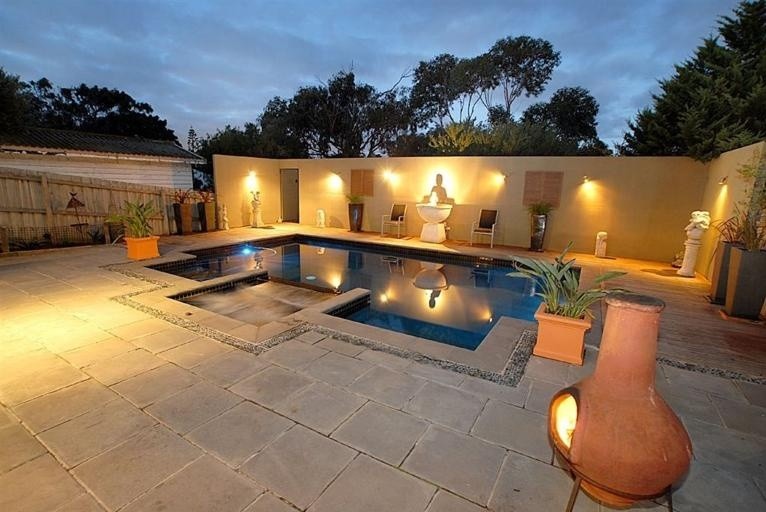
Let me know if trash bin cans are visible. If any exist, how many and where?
[109,225,125,243]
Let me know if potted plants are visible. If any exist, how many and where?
[509,241,628,367]
[346,193,365,232]
[105,196,162,261]
[709,209,766,322]
[173,187,217,235]
[528,201,553,252]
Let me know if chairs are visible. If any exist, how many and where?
[471,209,500,249]
[382,200,408,239]
[472,266,493,290]
[383,256,404,274]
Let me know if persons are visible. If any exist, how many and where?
[684,211,710,231]
